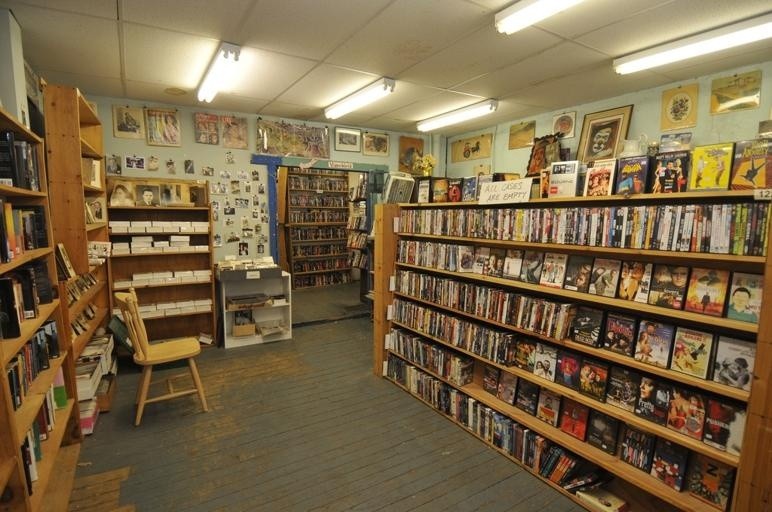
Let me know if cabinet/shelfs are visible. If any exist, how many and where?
[220,269,292,347]
[370,186,768,510]
[106,168,216,356]
[278,161,356,290]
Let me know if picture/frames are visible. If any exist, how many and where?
[362,132,390,156]
[334,126,362,153]
[576,104,636,164]
[526,132,560,179]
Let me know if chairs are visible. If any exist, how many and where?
[116,282,210,428]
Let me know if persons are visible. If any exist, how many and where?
[124,112,137,127]
[107,158,121,173]
[236,312,242,324]
[94,201,102,219]
[112,185,133,206]
[588,123,612,154]
[241,312,251,324]
[147,114,164,143]
[164,114,177,144]
[211,169,270,254]
[196,121,218,145]
[124,154,195,175]
[222,122,247,148]
[136,188,159,208]
[90,203,96,217]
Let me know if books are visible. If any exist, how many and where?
[392,270,758,393]
[199,330,213,346]
[55,239,119,434]
[396,239,764,327]
[109,220,212,320]
[82,157,101,188]
[397,202,768,256]
[288,166,367,288]
[271,296,286,304]
[0,122,68,496]
[393,298,748,458]
[389,323,735,511]
[215,254,278,271]
[387,141,772,201]
